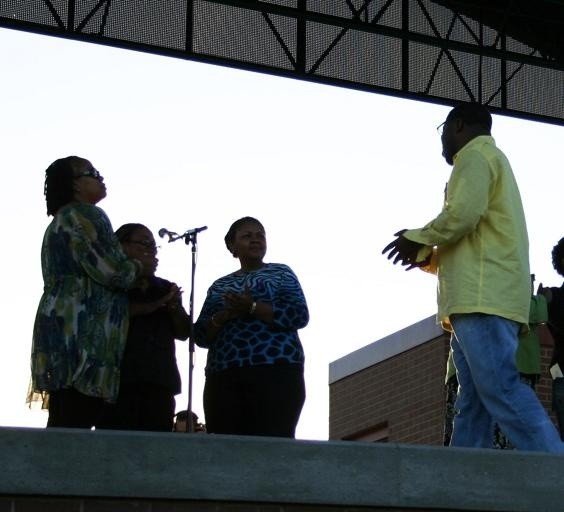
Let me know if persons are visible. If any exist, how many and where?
[115,221,193,432]
[442,273,554,450]
[192,216,310,438]
[30,156,159,428]
[537,237,564,441]
[381,101,564,455]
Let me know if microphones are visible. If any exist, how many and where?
[159,227,176,239]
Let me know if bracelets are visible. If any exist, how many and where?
[210,313,222,328]
[133,257,144,278]
[249,301,257,314]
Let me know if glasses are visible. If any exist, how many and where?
[127,239,152,248]
[73,170,100,178]
[437,120,452,134]
